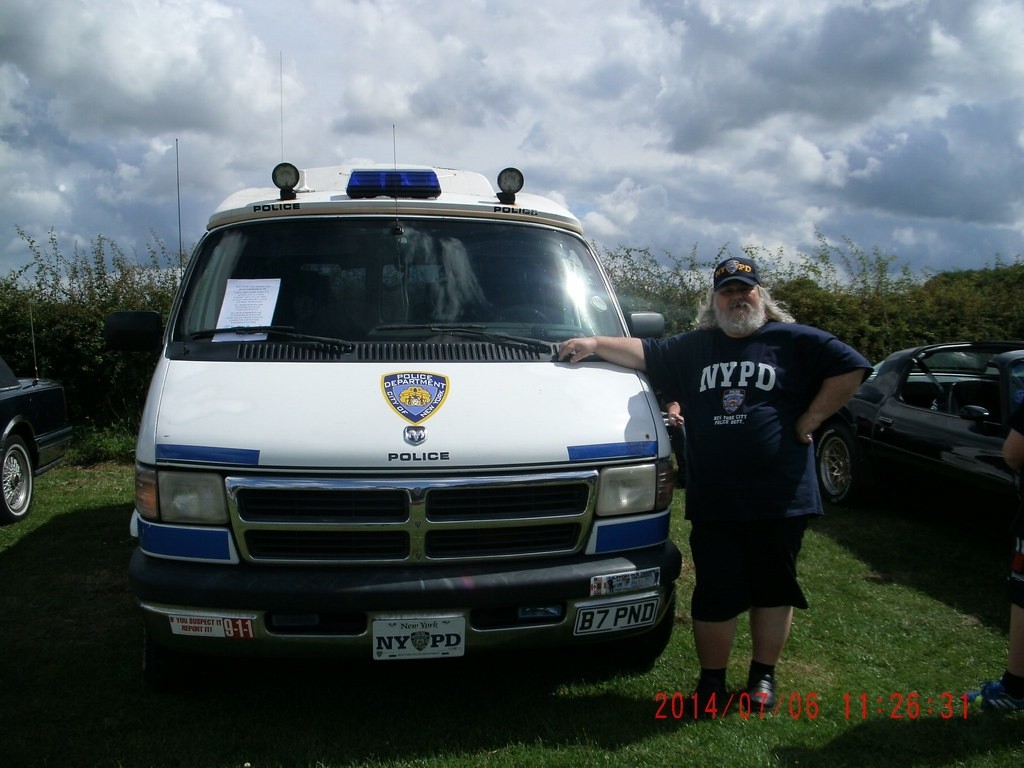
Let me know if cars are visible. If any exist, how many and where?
[815,340,1024,553]
[1,359,75,525]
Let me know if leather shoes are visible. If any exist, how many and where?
[742,675,779,713]
[677,687,734,721]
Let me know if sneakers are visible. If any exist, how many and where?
[961,678,1024,716]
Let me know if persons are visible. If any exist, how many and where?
[556,256,875,719]
[960,390,1024,711]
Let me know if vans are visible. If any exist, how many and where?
[120,162,681,698]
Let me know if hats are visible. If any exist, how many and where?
[711,257,763,293]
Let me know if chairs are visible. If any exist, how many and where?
[906,381,1000,423]
[456,256,544,323]
[265,270,368,341]
